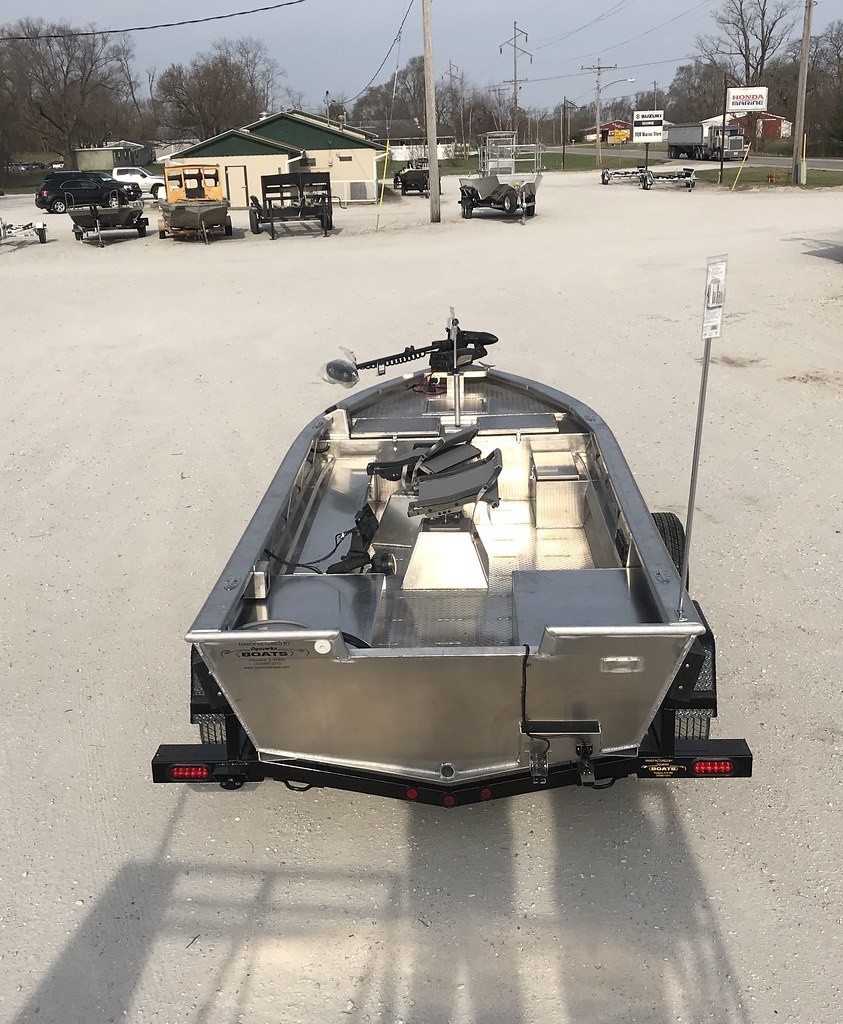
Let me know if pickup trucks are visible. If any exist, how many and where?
[89,166,166,199]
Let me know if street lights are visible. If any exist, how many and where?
[595,78,636,165]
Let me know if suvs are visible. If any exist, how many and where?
[35,170,143,213]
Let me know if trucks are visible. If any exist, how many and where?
[667,122,750,161]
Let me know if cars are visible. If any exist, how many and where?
[4,160,64,171]
